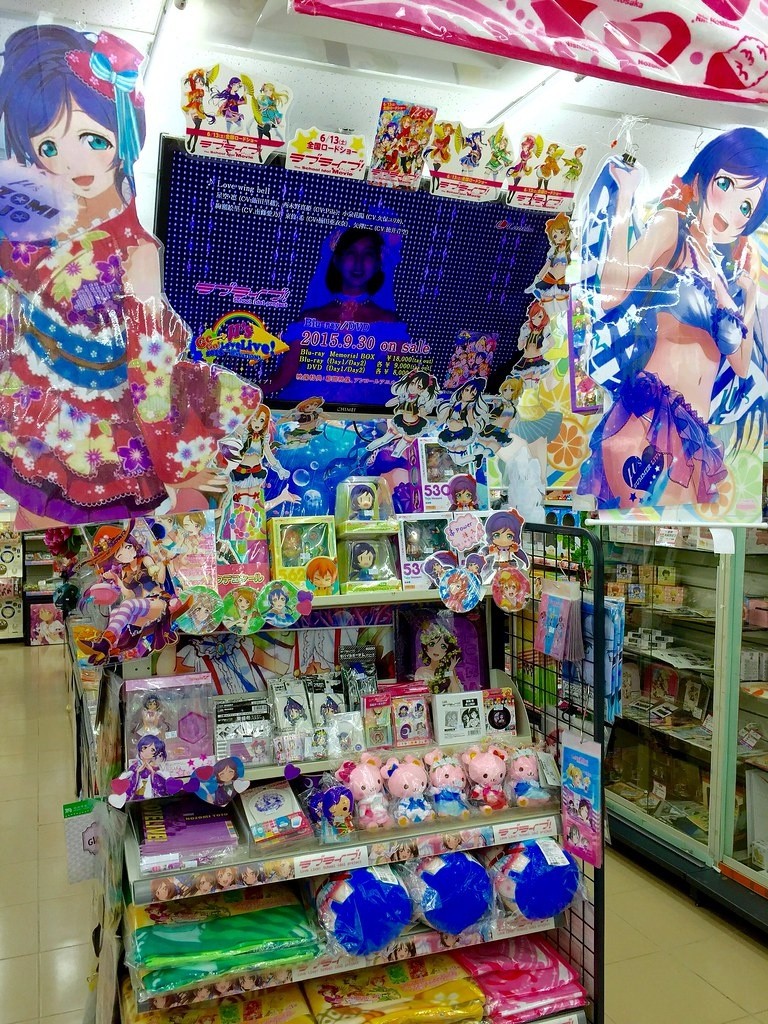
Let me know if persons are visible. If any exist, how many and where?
[132,694,170,738]
[300,225,403,323]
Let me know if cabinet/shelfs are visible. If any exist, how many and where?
[1,511,768,1024]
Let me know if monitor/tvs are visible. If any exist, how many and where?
[154,131,574,420]
